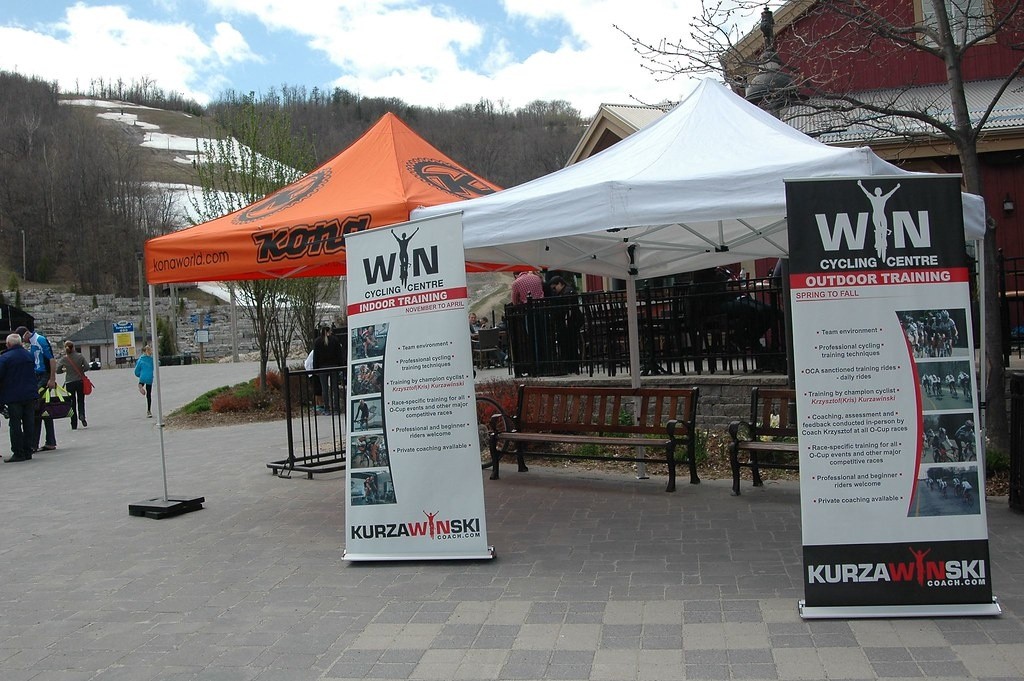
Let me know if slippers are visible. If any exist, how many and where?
[37,446,56,451]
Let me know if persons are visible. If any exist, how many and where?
[304,327,344,416]
[56,340,90,430]
[922,371,971,402]
[0,333,41,462]
[922,420,977,463]
[511,270,544,306]
[352,325,378,359]
[687,267,783,355]
[548,275,584,376]
[497,314,506,329]
[477,316,489,329]
[966,256,981,349]
[901,310,958,358]
[14,326,57,453]
[364,476,378,502]
[354,400,369,430]
[470,312,511,367]
[354,436,385,466]
[358,361,382,391]
[926,478,974,506]
[313,325,322,337]
[772,259,789,286]
[134,344,161,418]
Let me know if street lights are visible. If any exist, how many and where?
[743,2,810,433]
[135,251,147,348]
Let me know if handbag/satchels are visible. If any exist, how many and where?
[38,384,74,418]
[83,376,95,395]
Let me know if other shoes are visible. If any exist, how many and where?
[335,411,339,415]
[138,384,145,395]
[4,450,25,462]
[147,411,152,418]
[322,410,331,416]
[80,415,87,427]
[25,450,33,460]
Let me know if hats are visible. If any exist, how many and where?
[15,327,28,339]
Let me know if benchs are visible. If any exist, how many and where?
[488,383,702,493]
[726,388,798,494]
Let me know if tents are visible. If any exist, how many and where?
[128,103,548,521]
[410,76,986,506]
